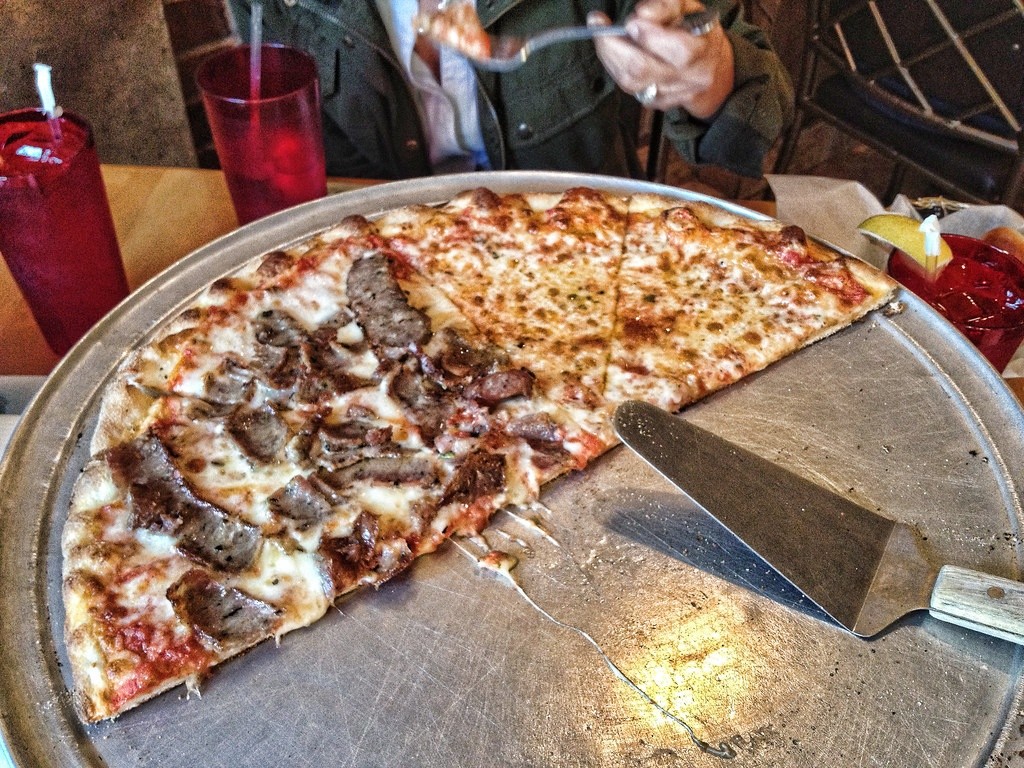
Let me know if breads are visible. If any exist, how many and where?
[979,226,1024,265]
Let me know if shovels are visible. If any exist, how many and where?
[611,399,1023,650]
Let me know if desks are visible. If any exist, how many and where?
[1,166,407,376]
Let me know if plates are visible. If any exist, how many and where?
[0,169,1024,768]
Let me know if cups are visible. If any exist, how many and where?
[0,101,133,361]
[197,42,328,233]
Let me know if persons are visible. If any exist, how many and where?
[226,0,796,202]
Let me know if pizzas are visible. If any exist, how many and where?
[61,183,904,723]
[411,0,490,61]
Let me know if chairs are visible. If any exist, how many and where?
[763,0,1024,207]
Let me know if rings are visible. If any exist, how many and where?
[637,84,657,105]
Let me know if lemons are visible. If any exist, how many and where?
[855,215,952,281]
